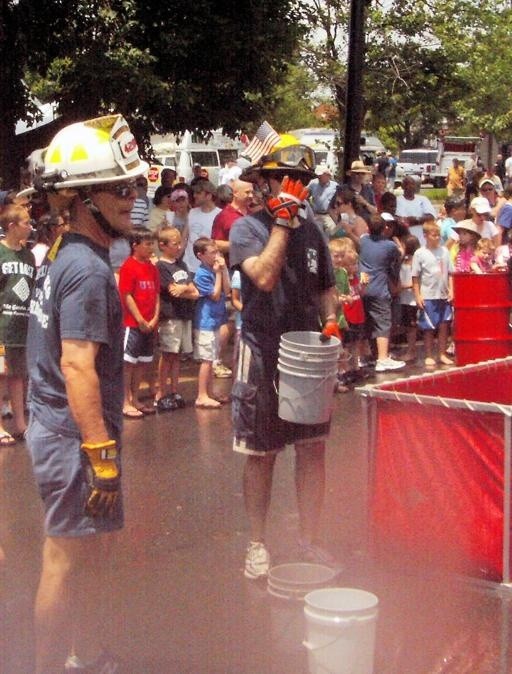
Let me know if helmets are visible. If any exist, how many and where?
[16,114,152,197]
[239,134,321,184]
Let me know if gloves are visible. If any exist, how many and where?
[318,318,346,346]
[76,438,123,523]
[261,174,311,229]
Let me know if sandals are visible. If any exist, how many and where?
[12,429,29,441]
[210,395,232,404]
[194,397,224,410]
[0,434,18,446]
[336,381,350,394]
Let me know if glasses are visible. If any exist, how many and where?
[20,203,34,210]
[333,201,347,208]
[266,171,312,188]
[85,176,139,199]
[480,188,494,193]
[352,172,367,176]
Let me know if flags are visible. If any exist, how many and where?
[240,120,280,167]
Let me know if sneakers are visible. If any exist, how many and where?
[212,362,233,379]
[446,342,455,356]
[355,367,374,379]
[373,356,407,373]
[152,393,185,412]
[242,538,274,582]
[289,539,348,578]
[339,374,368,391]
[62,643,135,674]
[0,401,13,419]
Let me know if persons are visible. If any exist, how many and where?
[23,113,150,673]
[231,133,344,581]
[1,150,512,449]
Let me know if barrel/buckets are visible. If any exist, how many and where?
[273,332,341,425]
[447,271,512,366]
[266,563,333,674]
[303,587,380,674]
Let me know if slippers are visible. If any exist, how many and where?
[123,409,145,419]
[138,404,157,416]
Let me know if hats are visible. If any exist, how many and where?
[344,161,373,179]
[469,197,492,216]
[380,211,399,226]
[478,179,496,190]
[450,219,482,239]
[192,162,203,170]
[169,188,190,203]
[191,180,218,197]
[313,165,334,178]
